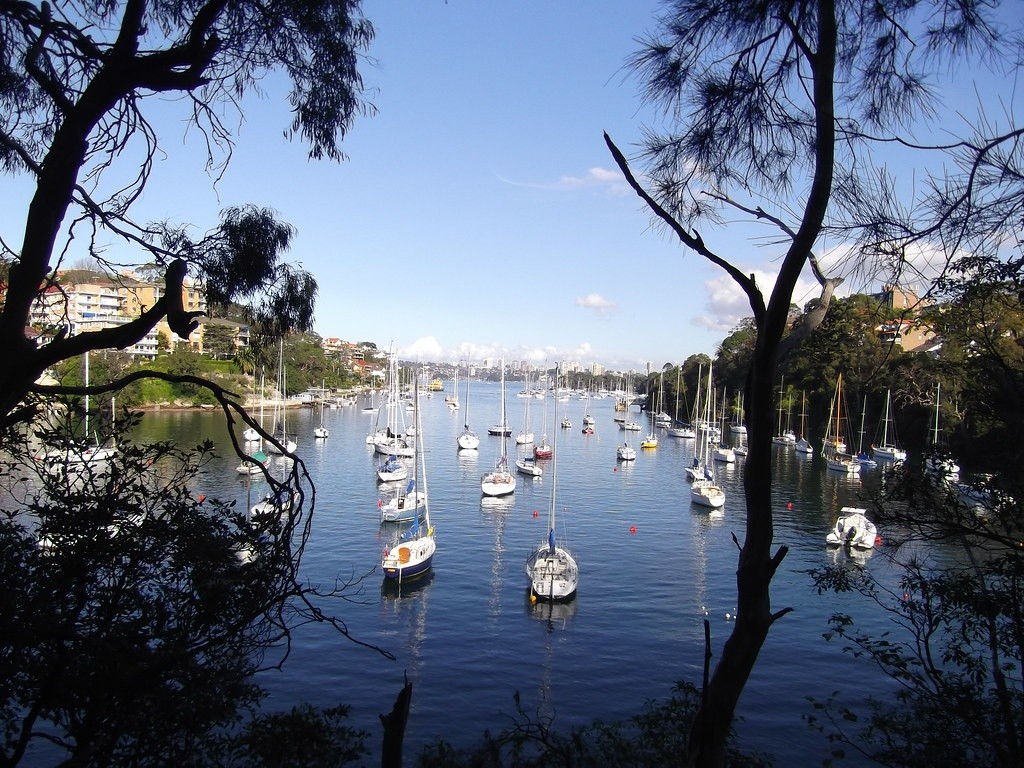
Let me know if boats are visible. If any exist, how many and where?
[824,507,877,549]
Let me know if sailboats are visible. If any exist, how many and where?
[487,357,513,437]
[819,371,862,472]
[515,356,543,476]
[382,375,436,581]
[640,392,659,449]
[224,435,259,569]
[381,374,427,523]
[242,336,459,472]
[481,353,516,497]
[456,351,482,450]
[525,361,579,601]
[252,386,299,516]
[852,394,877,466]
[515,363,535,444]
[923,381,961,473]
[870,388,908,462]
[616,374,637,461]
[533,357,552,459]
[517,358,849,482]
[236,362,273,475]
[690,360,726,509]
[39,349,119,464]
